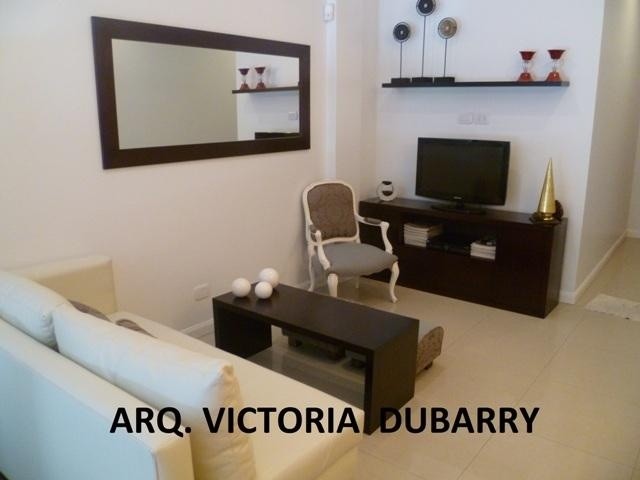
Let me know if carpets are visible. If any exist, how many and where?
[583,292,640,324]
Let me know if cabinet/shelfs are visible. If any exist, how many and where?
[358,195,569,321]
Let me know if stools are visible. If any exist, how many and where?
[281,293,445,377]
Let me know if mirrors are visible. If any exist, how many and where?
[89,13,313,173]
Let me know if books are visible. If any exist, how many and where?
[402,221,444,248]
[468,234,496,260]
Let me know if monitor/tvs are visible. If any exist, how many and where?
[415,136,510,214]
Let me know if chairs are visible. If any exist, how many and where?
[300,177,403,305]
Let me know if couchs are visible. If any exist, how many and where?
[0,251,368,480]
